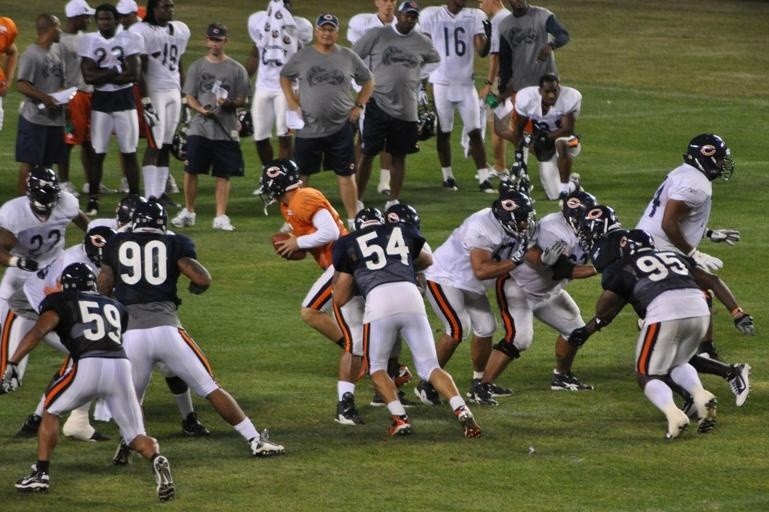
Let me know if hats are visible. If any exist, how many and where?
[65,0,96,18]
[116,0,138,15]
[206,22,228,41]
[396,0,420,15]
[315,13,340,31]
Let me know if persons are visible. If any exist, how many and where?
[98,202,286,463]
[589,133,740,362]
[171,23,250,230]
[414,191,565,407]
[243,1,581,196]
[334,199,480,437]
[467,192,597,396]
[254,158,348,350]
[2,262,174,501]
[1,166,210,435]
[16,0,191,215]
[569,205,754,439]
[1,16,17,97]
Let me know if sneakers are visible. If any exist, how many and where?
[59,174,236,232]
[376,168,580,215]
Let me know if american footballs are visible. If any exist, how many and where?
[272,233,306,261]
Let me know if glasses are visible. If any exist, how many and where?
[318,28,336,33]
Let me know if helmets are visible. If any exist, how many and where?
[353,207,385,232]
[497,176,534,197]
[171,134,191,161]
[24,167,164,261]
[61,262,99,291]
[562,191,658,257]
[252,158,303,205]
[491,190,535,239]
[418,108,436,141]
[383,204,420,229]
[237,112,254,138]
[683,133,735,182]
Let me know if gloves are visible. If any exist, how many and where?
[0,362,22,394]
[689,246,724,273]
[734,309,755,335]
[142,101,159,127]
[567,326,589,348]
[540,240,566,266]
[508,236,528,264]
[10,256,39,273]
[705,229,740,246]
[511,120,550,176]
[593,250,615,275]
